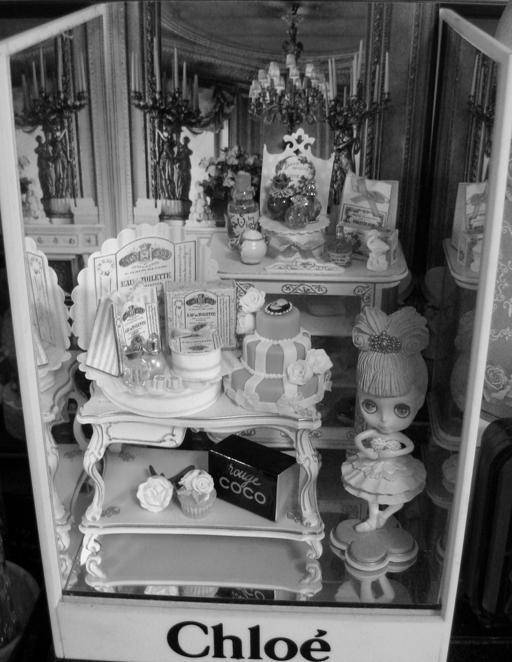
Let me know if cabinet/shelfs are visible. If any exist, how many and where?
[73,386,323,603]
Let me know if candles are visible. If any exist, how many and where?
[324,36,390,103]
[125,33,201,115]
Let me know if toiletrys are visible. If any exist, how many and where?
[328,226,354,267]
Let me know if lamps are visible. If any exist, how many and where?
[248,4,328,124]
[16,35,83,102]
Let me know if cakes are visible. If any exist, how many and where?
[223,306,322,411]
[176,468,218,519]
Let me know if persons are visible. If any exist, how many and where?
[340,306,430,533]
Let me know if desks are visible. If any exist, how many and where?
[208,228,409,458]
